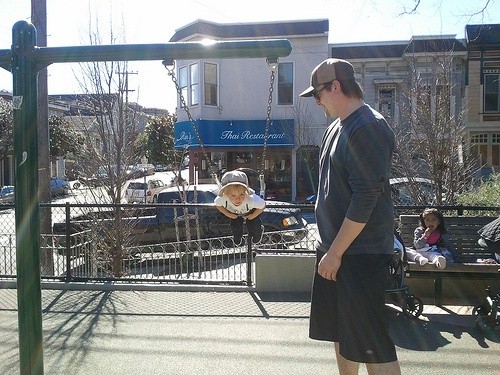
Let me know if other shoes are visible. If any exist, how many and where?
[253,225,264,243]
[234,238,242,245]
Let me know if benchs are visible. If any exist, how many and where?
[399,214,500,307]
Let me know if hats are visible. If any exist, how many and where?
[299,58,354,97]
[220,171,255,197]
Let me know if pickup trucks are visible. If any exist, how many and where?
[52,184,309,273]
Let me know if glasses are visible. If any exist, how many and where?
[312,83,331,99]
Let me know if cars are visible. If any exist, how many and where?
[51,176,70,198]
[389,176,459,215]
[93,163,172,186]
[0,185,14,211]
[124,178,168,205]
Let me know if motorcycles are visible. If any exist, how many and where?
[73,174,98,189]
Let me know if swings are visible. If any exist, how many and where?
[160,57,279,220]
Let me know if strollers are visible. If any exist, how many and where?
[473,217,500,335]
[385,230,424,320]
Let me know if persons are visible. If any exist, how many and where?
[299,58,402,375]
[405,208,454,270]
[213,171,266,246]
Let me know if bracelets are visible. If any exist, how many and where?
[424,233,428,238]
